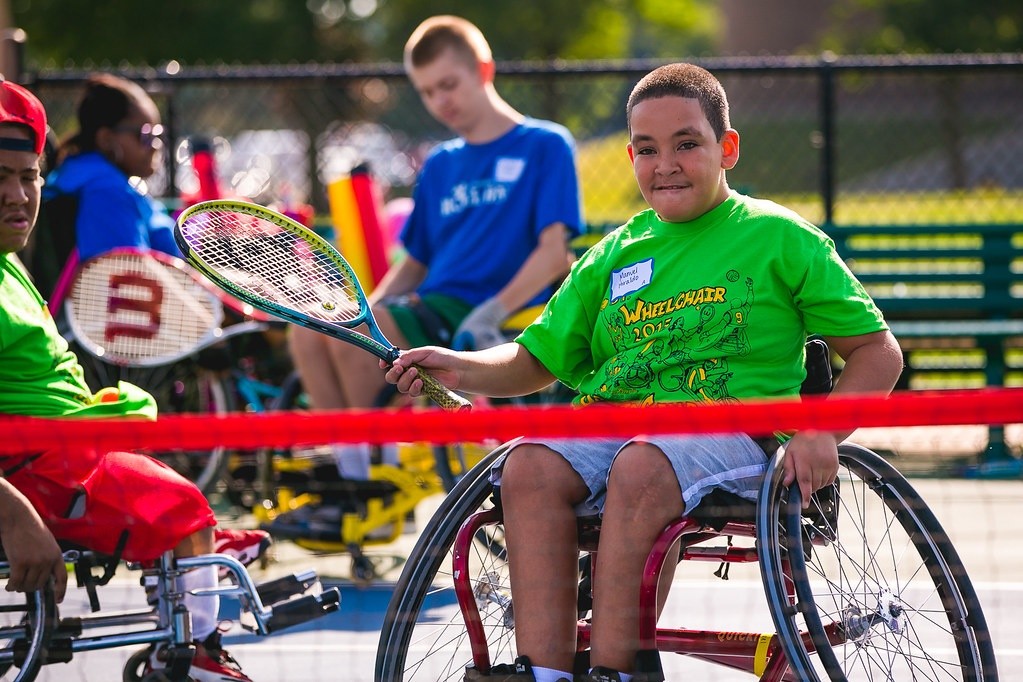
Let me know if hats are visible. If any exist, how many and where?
[0,74,47,155]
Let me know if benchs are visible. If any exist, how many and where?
[570,220,1023,479]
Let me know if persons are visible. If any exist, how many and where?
[1,81,250,682]
[378,63,904,682]
[26,16,585,543]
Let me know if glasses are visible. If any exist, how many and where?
[103,124,160,146]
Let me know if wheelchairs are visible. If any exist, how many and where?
[374,332,999,682]
[52,300,506,563]
[0,457,344,682]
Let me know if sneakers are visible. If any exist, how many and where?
[145,631,252,682]
[141,529,273,605]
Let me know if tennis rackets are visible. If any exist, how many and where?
[175,198,474,415]
[64,245,421,367]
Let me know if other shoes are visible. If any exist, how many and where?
[287,464,417,552]
[255,491,327,537]
[576,648,665,682]
[463,655,573,682]
